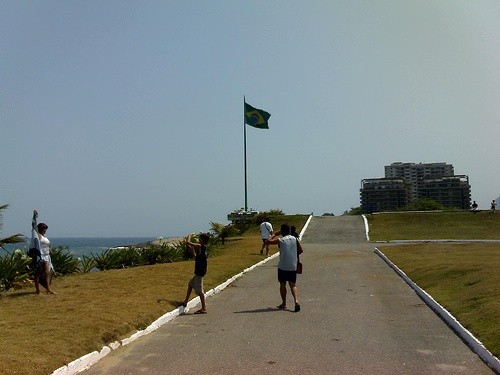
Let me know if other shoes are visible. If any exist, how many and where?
[47,289,58,295]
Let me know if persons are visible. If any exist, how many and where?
[259,218,275,257]
[267,224,303,311]
[180,234,209,314]
[29,209,58,295]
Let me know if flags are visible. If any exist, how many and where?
[244,102,271,129]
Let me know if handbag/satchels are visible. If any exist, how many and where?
[297,255,302,274]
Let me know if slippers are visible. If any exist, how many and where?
[177,301,187,307]
[295,305,300,312]
[277,304,287,308]
[194,310,207,314]
